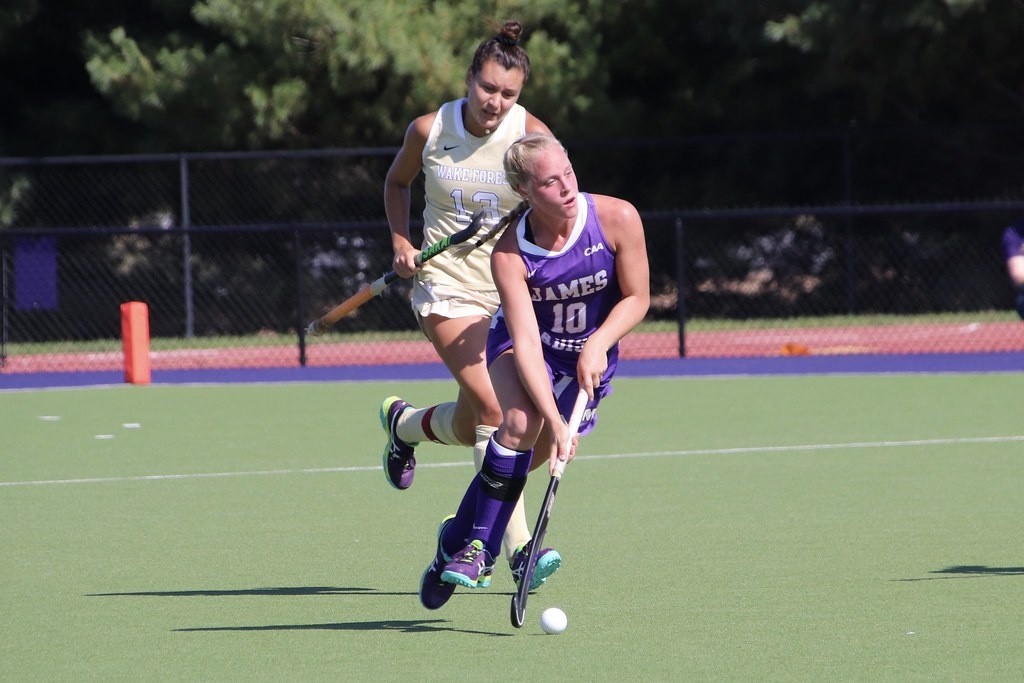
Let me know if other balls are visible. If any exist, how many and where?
[539,606,569,636]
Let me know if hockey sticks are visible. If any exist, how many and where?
[307,209,490,337]
[507,383,591,630]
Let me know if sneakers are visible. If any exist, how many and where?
[419,515,457,610]
[509,538,561,592]
[380,396,419,490]
[440,540,496,588]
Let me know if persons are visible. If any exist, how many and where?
[380,22,561,593]
[421,134,650,611]
[1003,221,1024,319]
